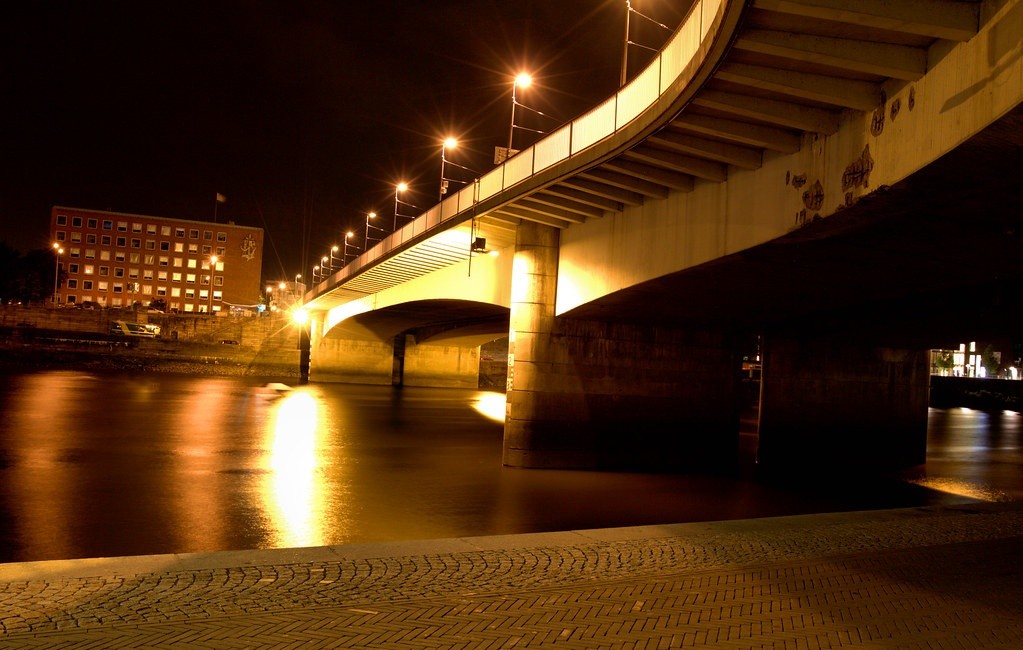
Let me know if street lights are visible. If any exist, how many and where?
[393,182,408,233]
[53,240,64,301]
[329,247,338,275]
[365,212,376,251]
[265,287,272,298]
[294,274,301,301]
[507,73,532,160]
[439,139,456,201]
[343,232,353,267]
[209,255,218,315]
[312,265,319,288]
[318,257,328,282]
[279,283,286,309]
